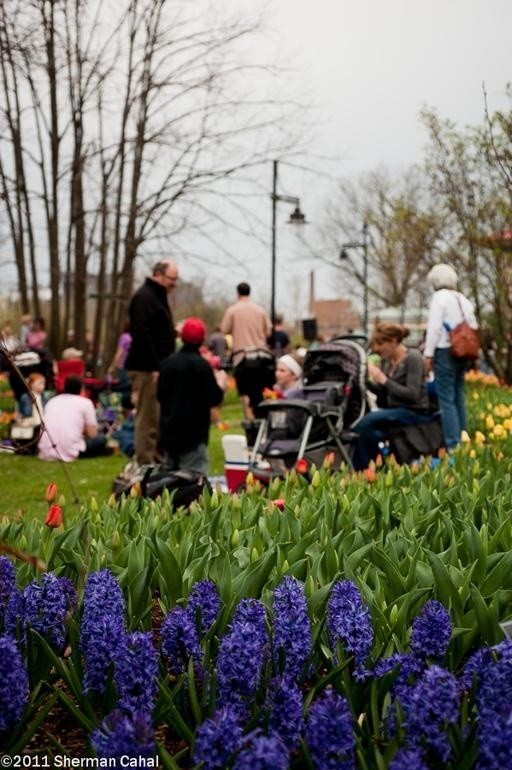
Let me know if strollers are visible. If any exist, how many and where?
[242,332,376,490]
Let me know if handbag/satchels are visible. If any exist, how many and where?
[391,418,445,464]
[451,320,482,359]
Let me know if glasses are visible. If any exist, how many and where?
[161,273,177,280]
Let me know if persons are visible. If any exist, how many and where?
[418,329,434,396]
[218,281,275,421]
[351,323,429,475]
[423,263,480,452]
[0,311,305,474]
[126,260,181,467]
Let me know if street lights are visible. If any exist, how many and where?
[268,158,311,332]
[340,218,370,332]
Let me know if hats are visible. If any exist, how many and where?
[182,317,206,346]
